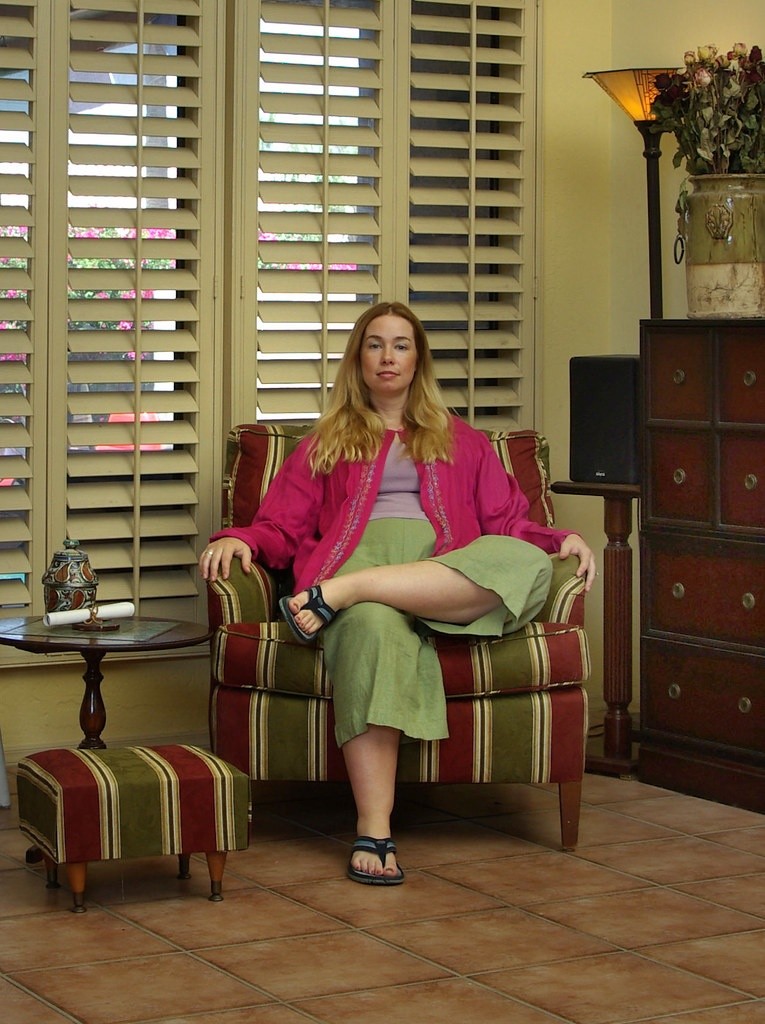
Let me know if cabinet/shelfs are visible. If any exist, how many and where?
[635,319,765,815]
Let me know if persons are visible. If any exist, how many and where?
[199,303,600,885]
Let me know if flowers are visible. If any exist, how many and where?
[643,43,765,237]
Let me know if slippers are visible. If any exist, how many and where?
[280,585,336,643]
[348,835,405,885]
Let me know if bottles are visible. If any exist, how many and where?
[42,538,99,615]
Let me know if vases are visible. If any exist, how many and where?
[673,173,765,320]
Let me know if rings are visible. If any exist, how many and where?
[595,571,599,576]
[204,551,213,556]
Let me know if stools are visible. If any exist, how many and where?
[15,744,255,914]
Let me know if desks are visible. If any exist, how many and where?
[0,616,215,749]
[549,481,641,783]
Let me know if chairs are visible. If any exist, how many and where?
[210,425,592,854]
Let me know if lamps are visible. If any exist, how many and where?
[580,67,683,320]
